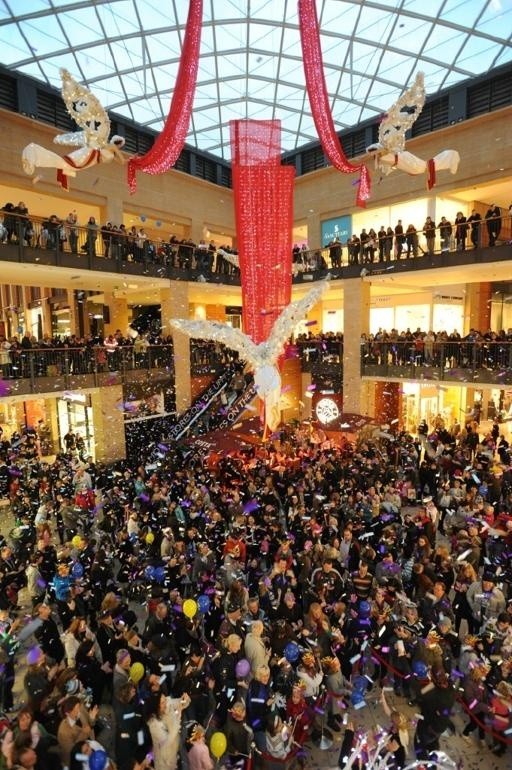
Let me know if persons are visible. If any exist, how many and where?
[1,199,512,769]
